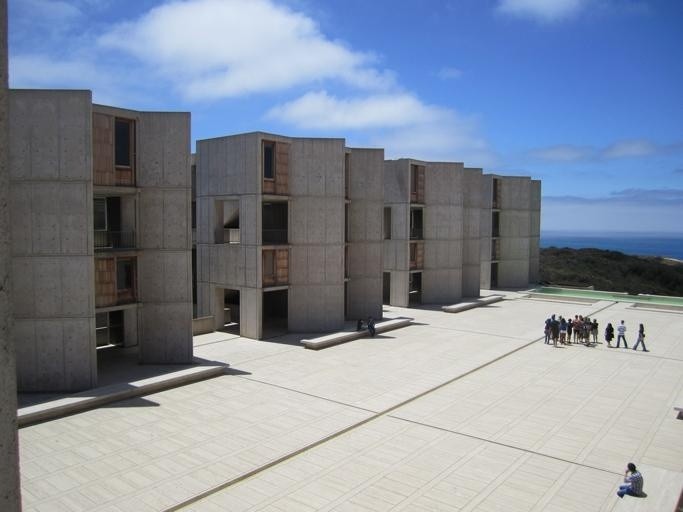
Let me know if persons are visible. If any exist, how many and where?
[617,462,643,498]
[541,312,601,347]
[357,318,365,331]
[603,322,614,347]
[617,320,628,349]
[366,317,375,337]
[632,323,647,353]
